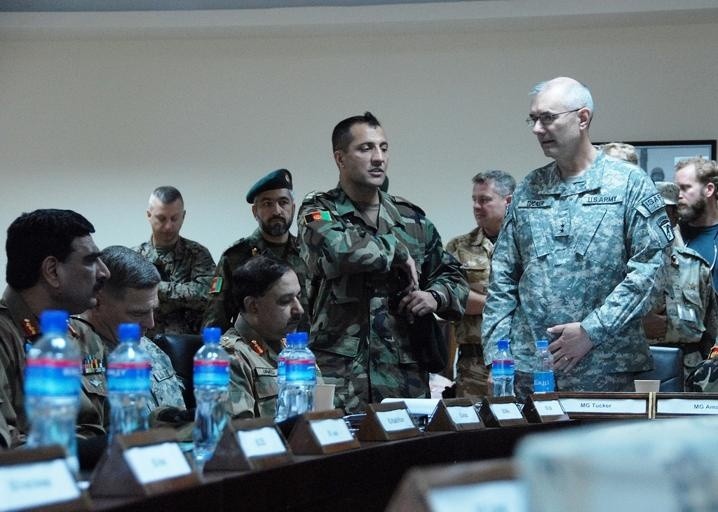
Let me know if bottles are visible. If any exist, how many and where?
[193,325,233,452]
[276,333,317,422]
[532,339,555,394]
[26,308,82,471]
[490,340,515,395]
[108,322,153,452]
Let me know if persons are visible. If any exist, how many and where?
[295,110,469,412]
[447,169,516,397]
[1,167,308,451]
[601,144,717,392]
[479,76,672,395]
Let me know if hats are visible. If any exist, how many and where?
[655,181,680,205]
[247,168,291,204]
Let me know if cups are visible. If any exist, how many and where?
[631,378,662,392]
[312,384,336,415]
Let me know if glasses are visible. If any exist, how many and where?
[526,108,585,126]
